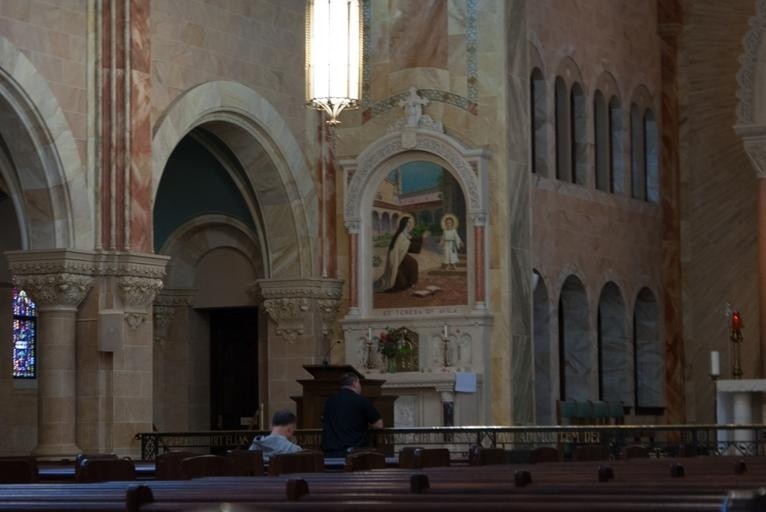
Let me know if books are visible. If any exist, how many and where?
[412,283,443,299]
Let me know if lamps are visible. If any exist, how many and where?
[301,0,367,129]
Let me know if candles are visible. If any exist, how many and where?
[732,311,743,329]
[710,347,722,376]
[368,326,372,343]
[444,322,449,340]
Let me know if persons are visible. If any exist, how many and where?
[246,407,304,467]
[436,213,463,272]
[370,214,432,296]
[317,371,382,471]
